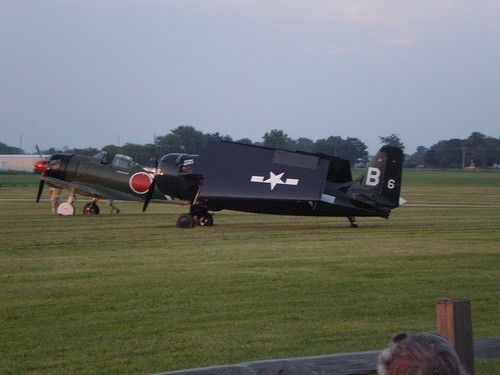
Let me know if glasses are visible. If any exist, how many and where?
[392,332,408,345]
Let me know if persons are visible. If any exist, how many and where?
[49,187,61,216]
[108,199,121,215]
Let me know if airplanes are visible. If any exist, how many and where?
[144,138,403,228]
[34,144,171,214]
